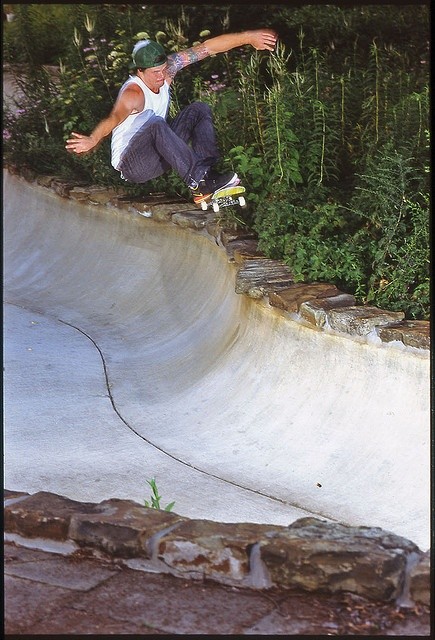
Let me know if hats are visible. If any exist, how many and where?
[128,40,167,69]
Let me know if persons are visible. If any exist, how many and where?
[65,28,278,206]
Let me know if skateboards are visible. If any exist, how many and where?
[201,185,246,212]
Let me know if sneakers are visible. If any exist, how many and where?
[188,171,240,208]
